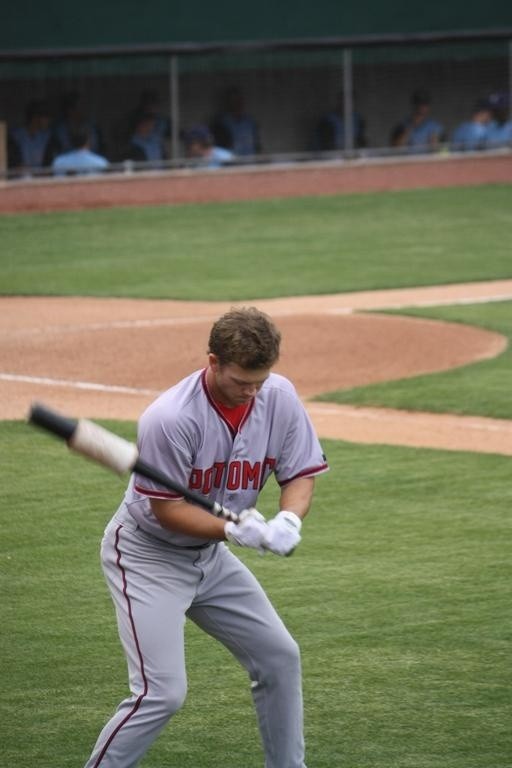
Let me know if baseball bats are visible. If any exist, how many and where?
[27,401,297,557]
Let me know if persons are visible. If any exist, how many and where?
[0,87,512,178]
[83,305,330,768]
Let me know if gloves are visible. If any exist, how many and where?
[224,506,270,549]
[258,510,303,558]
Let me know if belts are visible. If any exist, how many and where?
[189,543,210,551]
